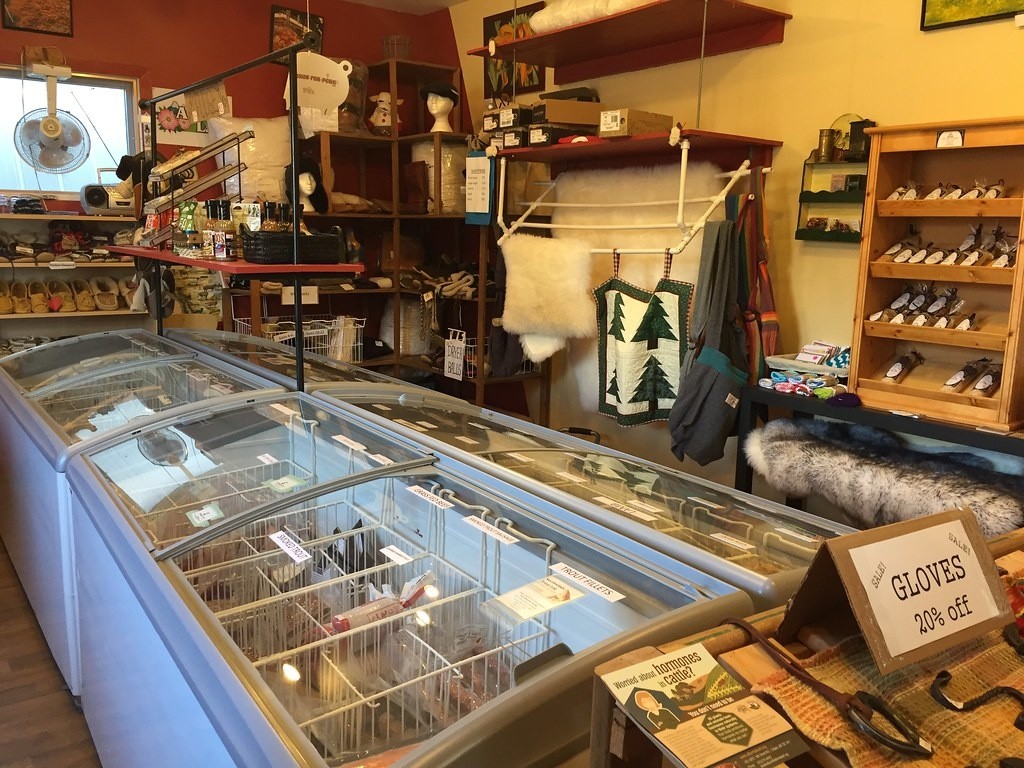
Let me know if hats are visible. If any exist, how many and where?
[285,158,329,214]
[420,80,459,108]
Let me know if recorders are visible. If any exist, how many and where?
[80,168,135,218]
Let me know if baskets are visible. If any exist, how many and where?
[7,337,51,353]
[234,314,366,365]
[239,223,342,264]
[445,328,536,378]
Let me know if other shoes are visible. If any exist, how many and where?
[0,220,134,263]
[0,276,147,314]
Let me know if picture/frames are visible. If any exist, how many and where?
[0,0,74,38]
[920,0,1024,32]
[268,3,325,68]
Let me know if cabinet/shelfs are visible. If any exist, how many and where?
[0,59,549,427]
[467,0,793,181]
[794,147,868,243]
[847,115,1024,432]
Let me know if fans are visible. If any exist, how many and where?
[14,62,92,174]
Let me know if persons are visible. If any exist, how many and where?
[285,157,328,214]
[420,82,460,132]
